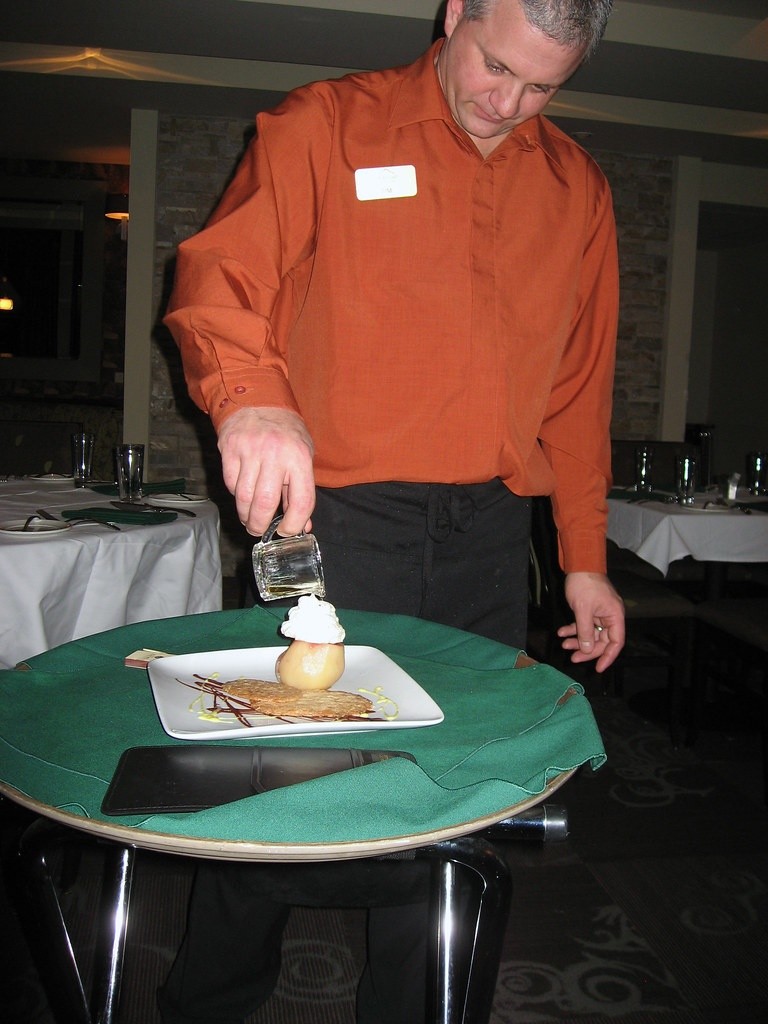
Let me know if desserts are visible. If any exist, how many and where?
[280,597,344,689]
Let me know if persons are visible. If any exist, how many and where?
[102,222,125,356]
[161,1,625,1024]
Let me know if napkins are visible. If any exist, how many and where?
[734,502,768,513]
[606,487,670,504]
[93,479,186,496]
[662,486,705,493]
[60,508,177,524]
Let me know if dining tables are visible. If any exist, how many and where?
[0,604,601,1024]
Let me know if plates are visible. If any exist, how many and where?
[148,493,209,504]
[29,476,74,480]
[681,503,730,513]
[147,645,446,741]
[0,519,72,537]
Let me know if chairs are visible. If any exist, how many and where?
[605,439,703,488]
[692,593,768,756]
[0,422,82,475]
[525,495,700,751]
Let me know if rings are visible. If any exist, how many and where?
[595,625,603,631]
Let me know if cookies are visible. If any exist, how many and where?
[252,689,373,716]
[224,679,303,701]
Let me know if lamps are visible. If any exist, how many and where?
[0,276,23,314]
[100,193,133,221]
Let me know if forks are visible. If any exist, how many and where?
[36,509,81,523]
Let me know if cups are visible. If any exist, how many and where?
[72,433,93,488]
[749,452,768,496]
[634,446,655,499]
[676,456,696,506]
[251,515,326,602]
[116,444,144,503]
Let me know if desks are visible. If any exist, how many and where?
[599,483,768,708]
[0,462,222,674]
